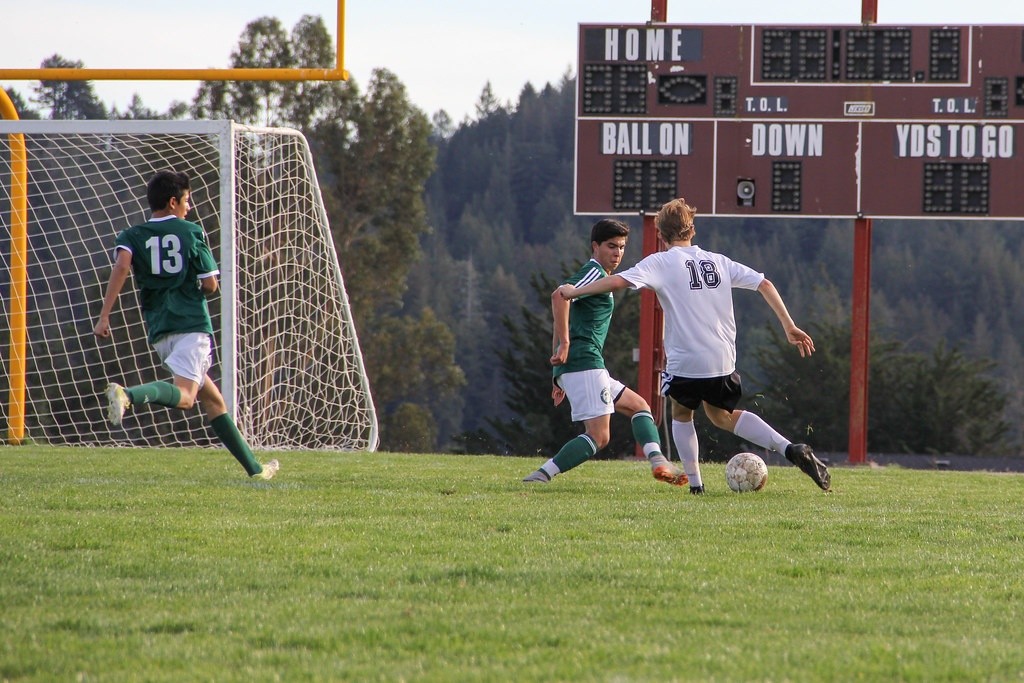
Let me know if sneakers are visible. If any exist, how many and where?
[524,476,548,486]
[651,460,689,486]
[792,444,830,490]
[104,382,131,427]
[252,459,279,482]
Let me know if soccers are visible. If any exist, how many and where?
[726,453,768,493]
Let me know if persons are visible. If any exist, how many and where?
[91,169,280,482]
[559,197,831,492]
[520,219,689,486]
